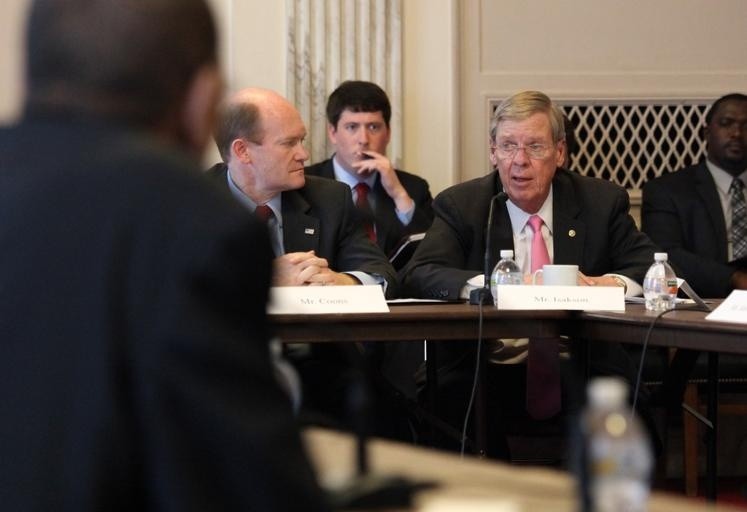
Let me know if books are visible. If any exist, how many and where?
[388,227,428,270]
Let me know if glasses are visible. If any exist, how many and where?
[493,142,556,159]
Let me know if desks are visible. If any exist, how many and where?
[268,298,746,505]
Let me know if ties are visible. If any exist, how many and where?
[256,205,277,257]
[528,216,562,416]
[730,178,747,262]
[353,182,375,243]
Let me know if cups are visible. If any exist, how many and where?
[532,265,580,286]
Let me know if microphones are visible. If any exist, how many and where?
[470,192,509,303]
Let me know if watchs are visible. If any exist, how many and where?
[608,274,626,289]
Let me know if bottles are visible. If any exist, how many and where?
[490,249,522,308]
[572,378,654,511]
[641,252,678,312]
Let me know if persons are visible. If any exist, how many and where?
[0,0,329,512]
[302,79,433,393]
[205,85,400,444]
[638,91,746,301]
[396,89,681,488]
